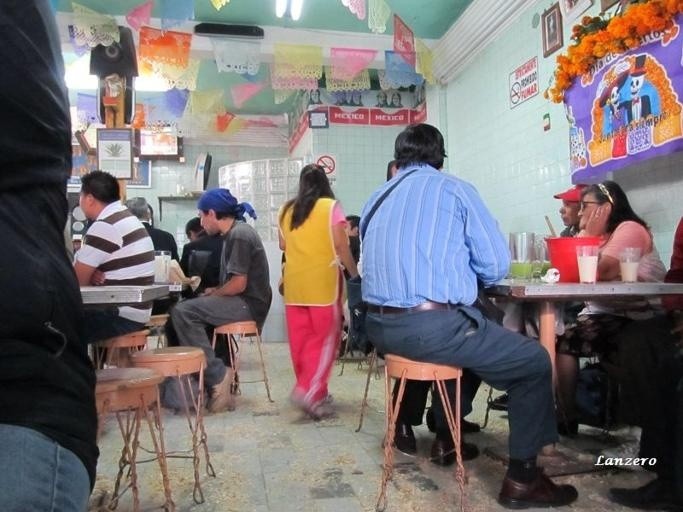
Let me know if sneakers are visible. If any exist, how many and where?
[208,367,235,413]
[489,394,510,411]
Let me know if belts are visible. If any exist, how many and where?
[366,300,462,317]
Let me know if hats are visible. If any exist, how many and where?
[553,184,589,201]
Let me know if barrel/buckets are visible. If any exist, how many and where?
[545,236,601,282]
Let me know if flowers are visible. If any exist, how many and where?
[570,14,613,42]
[545,0,683,103]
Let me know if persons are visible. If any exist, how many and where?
[277,165,356,417]
[359,123,587,508]
[165,187,272,416]
[307,90,323,106]
[71,235,83,264]
[391,92,403,107]
[334,90,347,105]
[167,218,240,395]
[551,182,666,437]
[123,197,181,315]
[72,172,157,342]
[486,182,589,412]
[0,0,99,511]
[601,214,683,510]
[341,213,366,352]
[375,92,388,107]
[350,92,363,105]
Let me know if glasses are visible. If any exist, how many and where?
[580,201,601,212]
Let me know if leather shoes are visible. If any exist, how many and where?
[609,477,682,511]
[431,418,480,434]
[555,411,578,437]
[430,440,479,467]
[498,466,578,509]
[395,424,417,455]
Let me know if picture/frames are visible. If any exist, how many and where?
[540,3,564,58]
[133,123,184,162]
[308,110,329,129]
[96,127,134,182]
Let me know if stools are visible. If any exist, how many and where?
[132,345,218,505]
[209,319,275,411]
[376,351,464,509]
[143,310,172,349]
[92,367,180,512]
[91,333,151,367]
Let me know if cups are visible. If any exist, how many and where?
[510,231,546,283]
[575,245,599,283]
[153,251,171,284]
[620,247,640,281]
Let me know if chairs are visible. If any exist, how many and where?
[341,279,376,373]
